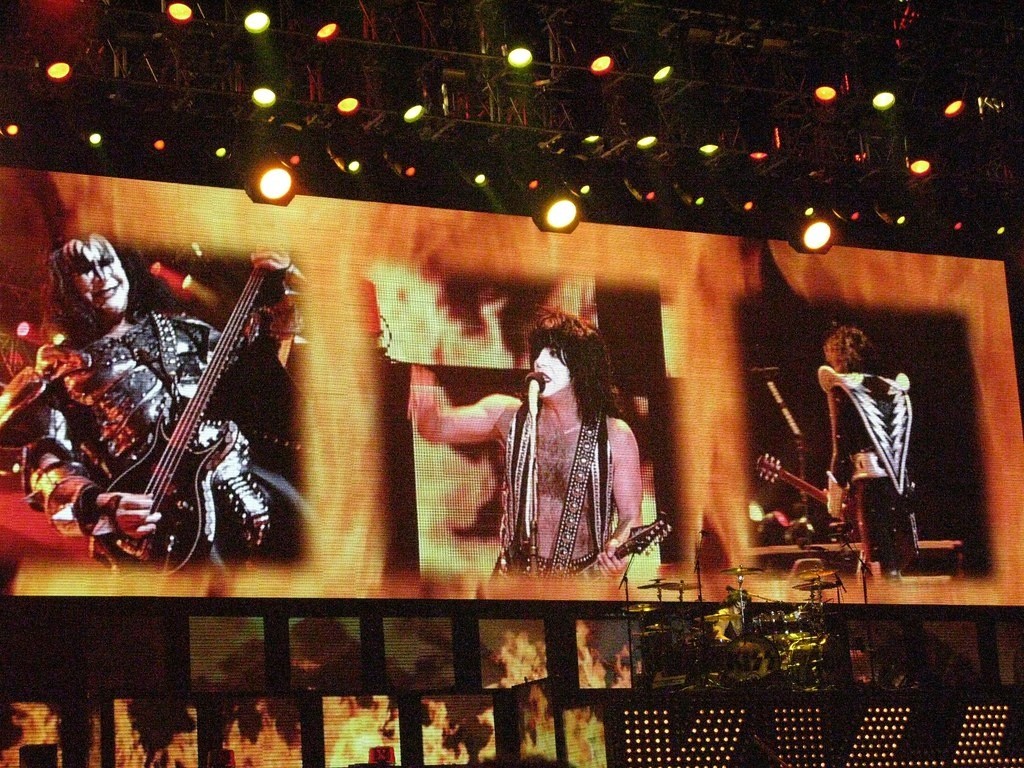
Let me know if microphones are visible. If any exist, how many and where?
[698,530,706,548]
[524,371,546,415]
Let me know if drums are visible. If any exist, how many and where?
[722,633,783,683]
[788,634,846,688]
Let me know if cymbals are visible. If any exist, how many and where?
[662,580,702,591]
[695,613,740,621]
[796,565,840,578]
[719,564,764,576]
[791,582,842,591]
[621,603,659,612]
[636,577,680,591]
[645,623,670,630]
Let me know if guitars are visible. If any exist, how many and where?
[754,451,856,527]
[92,264,269,576]
[577,510,673,585]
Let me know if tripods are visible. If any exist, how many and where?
[677,541,903,699]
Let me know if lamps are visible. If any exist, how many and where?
[0,0,1024,254]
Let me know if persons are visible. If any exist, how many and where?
[0,229,312,571]
[409,306,643,600]
[819,318,919,583]
[712,589,751,643]
